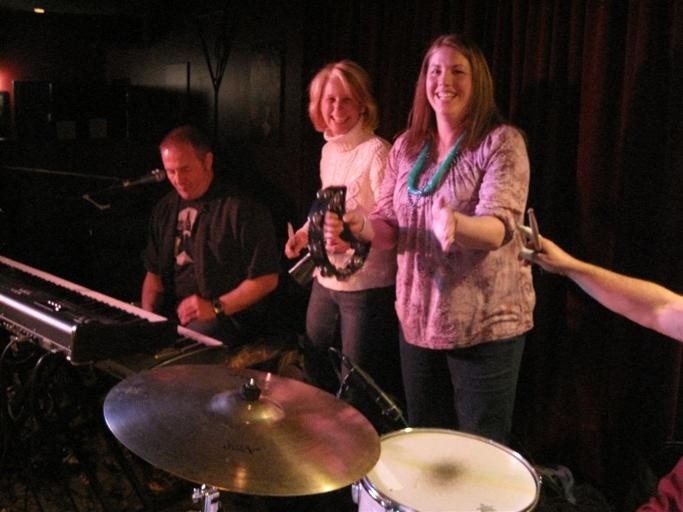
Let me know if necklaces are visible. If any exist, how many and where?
[405,130,482,196]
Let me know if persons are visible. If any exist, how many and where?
[323,32,536,440]
[140,125,283,336]
[513,226,683,512]
[283,59,397,382]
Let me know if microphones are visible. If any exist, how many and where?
[331,347,408,430]
[106,168,166,194]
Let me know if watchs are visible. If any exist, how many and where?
[210,295,225,320]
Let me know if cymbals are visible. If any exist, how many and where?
[103,364,381,497]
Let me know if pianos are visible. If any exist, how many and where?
[0,255,228,380]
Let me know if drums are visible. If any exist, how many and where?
[351,426,543,512]
